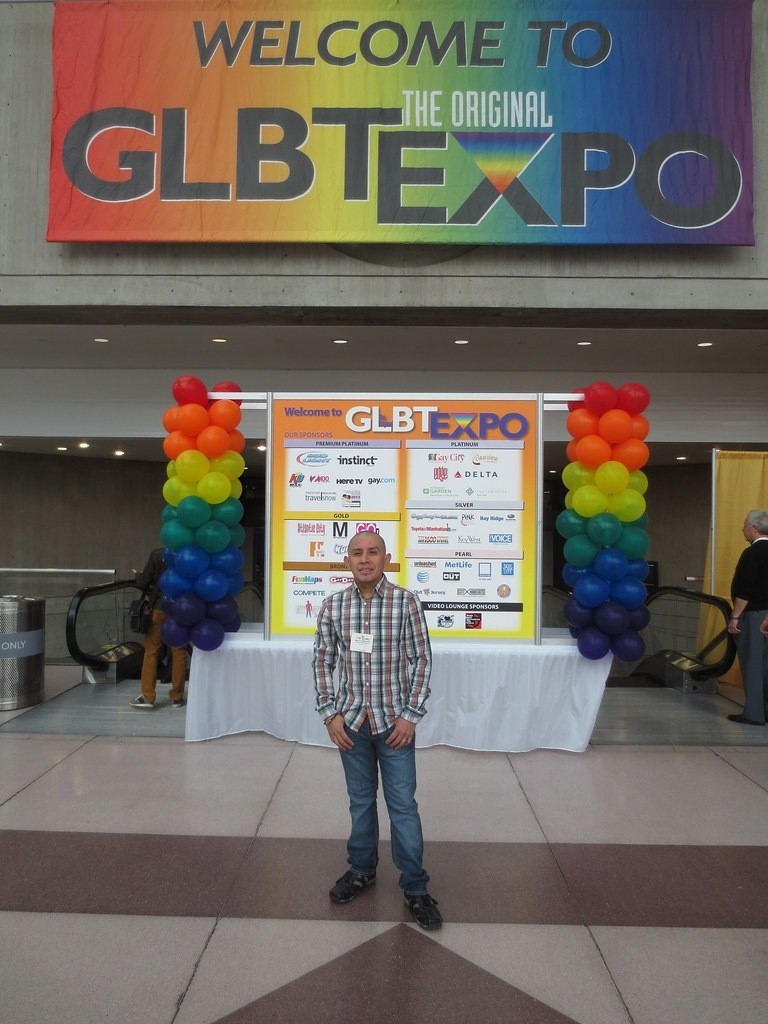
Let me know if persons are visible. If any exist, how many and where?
[129,547,187,708]
[314,531,444,931]
[727,509,768,725]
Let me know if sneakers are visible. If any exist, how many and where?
[404,893,442,929]
[171,698,184,706]
[330,871,377,903]
[129,696,153,707]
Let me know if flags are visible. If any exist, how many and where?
[698,451,768,688]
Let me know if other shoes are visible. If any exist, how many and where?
[728,715,764,726]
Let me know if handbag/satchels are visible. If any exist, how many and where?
[128,599,153,633]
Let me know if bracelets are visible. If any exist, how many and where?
[324,714,337,725]
[731,618,739,620]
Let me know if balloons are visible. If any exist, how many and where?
[159,376,245,651]
[557,382,650,660]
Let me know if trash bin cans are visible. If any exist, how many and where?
[0,592,48,712]
